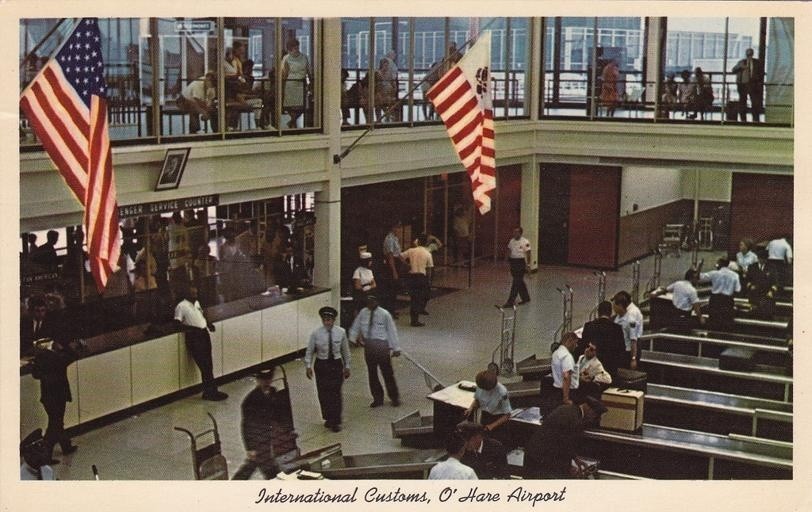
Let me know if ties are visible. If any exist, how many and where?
[327,330,335,362]
[368,310,374,338]
[747,60,752,82]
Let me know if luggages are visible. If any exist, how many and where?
[599,389,644,434]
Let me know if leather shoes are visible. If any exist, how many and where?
[418,309,430,315]
[324,419,343,433]
[371,401,386,408]
[391,399,400,407]
[201,390,229,401]
[62,445,78,456]
[411,321,427,327]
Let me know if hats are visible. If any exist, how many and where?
[20,428,43,454]
[585,395,608,415]
[318,306,337,319]
[365,292,378,302]
[360,251,373,260]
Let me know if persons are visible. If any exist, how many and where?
[448,41,465,66]
[453,209,474,268]
[545,331,581,409]
[612,294,642,373]
[660,72,677,119]
[419,58,445,121]
[18,430,56,480]
[600,58,618,117]
[348,255,376,348]
[578,300,626,372]
[731,48,763,124]
[18,210,317,310]
[690,66,714,119]
[454,421,510,480]
[20,293,69,356]
[741,298,779,320]
[159,152,192,192]
[678,71,696,117]
[175,283,229,401]
[575,339,613,402]
[413,233,443,316]
[736,237,761,282]
[232,364,290,481]
[747,250,781,307]
[303,307,351,435]
[399,236,433,330]
[503,227,534,307]
[345,296,403,409]
[694,255,741,330]
[173,36,408,138]
[31,327,83,460]
[661,270,707,333]
[768,234,792,280]
[383,220,406,319]
[618,289,644,357]
[430,432,479,480]
[525,396,609,483]
[465,372,515,452]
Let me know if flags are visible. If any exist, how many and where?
[425,30,500,214]
[18,16,122,297]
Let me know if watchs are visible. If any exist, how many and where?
[631,355,638,362]
